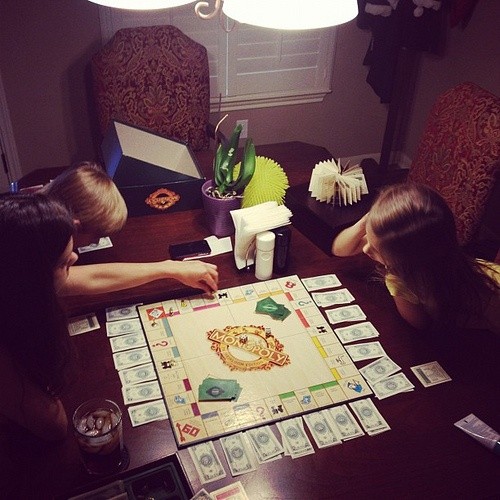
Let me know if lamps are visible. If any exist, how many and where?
[85,0,359,33]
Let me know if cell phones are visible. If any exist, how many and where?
[169,240,211,260]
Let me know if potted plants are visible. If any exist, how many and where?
[200,124,256,238]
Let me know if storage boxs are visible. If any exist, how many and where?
[100,118,206,217]
[284,182,380,257]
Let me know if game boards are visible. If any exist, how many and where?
[136,275,373,446]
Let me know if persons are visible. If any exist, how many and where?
[21,162,128,254]
[0,189,219,443]
[331,183,500,332]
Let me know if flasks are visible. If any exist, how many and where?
[255,231,275,280]
[272,227,291,274]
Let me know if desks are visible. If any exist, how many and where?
[0,141,499,500]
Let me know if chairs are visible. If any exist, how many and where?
[85,25,226,164]
[402,81,500,247]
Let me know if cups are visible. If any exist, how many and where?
[72,399,126,475]
